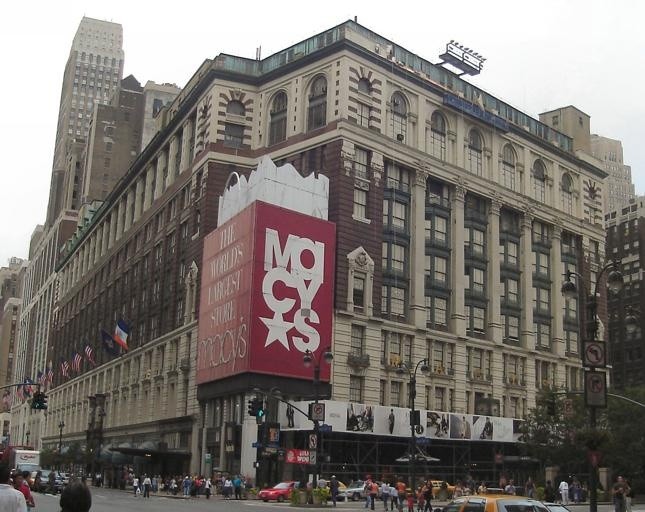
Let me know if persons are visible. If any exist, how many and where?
[284,402,295,428]
[348,404,372,432]
[222,475,246,499]
[388,408,396,434]
[479,415,490,438]
[295,476,337,506]
[453,479,515,496]
[526,474,633,511]
[183,474,212,499]
[134,474,182,498]
[1,444,103,511]
[458,415,467,438]
[364,477,434,512]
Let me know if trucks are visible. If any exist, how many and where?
[9,449,40,473]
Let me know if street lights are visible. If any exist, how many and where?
[303,343,333,489]
[396,357,432,498]
[24,431,30,449]
[560,258,624,512]
[57,421,64,469]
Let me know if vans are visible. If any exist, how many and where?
[59,472,69,483]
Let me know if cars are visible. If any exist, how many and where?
[258,480,304,502]
[543,503,571,512]
[345,480,388,501]
[27,471,37,490]
[433,494,551,512]
[405,479,455,500]
[476,481,504,494]
[506,486,524,496]
[306,480,346,502]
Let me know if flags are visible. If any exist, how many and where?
[11,318,130,396]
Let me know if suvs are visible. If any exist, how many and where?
[33,470,64,493]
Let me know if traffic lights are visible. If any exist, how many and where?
[247,398,256,416]
[564,398,574,415]
[31,393,40,409]
[38,393,47,410]
[256,400,264,417]
